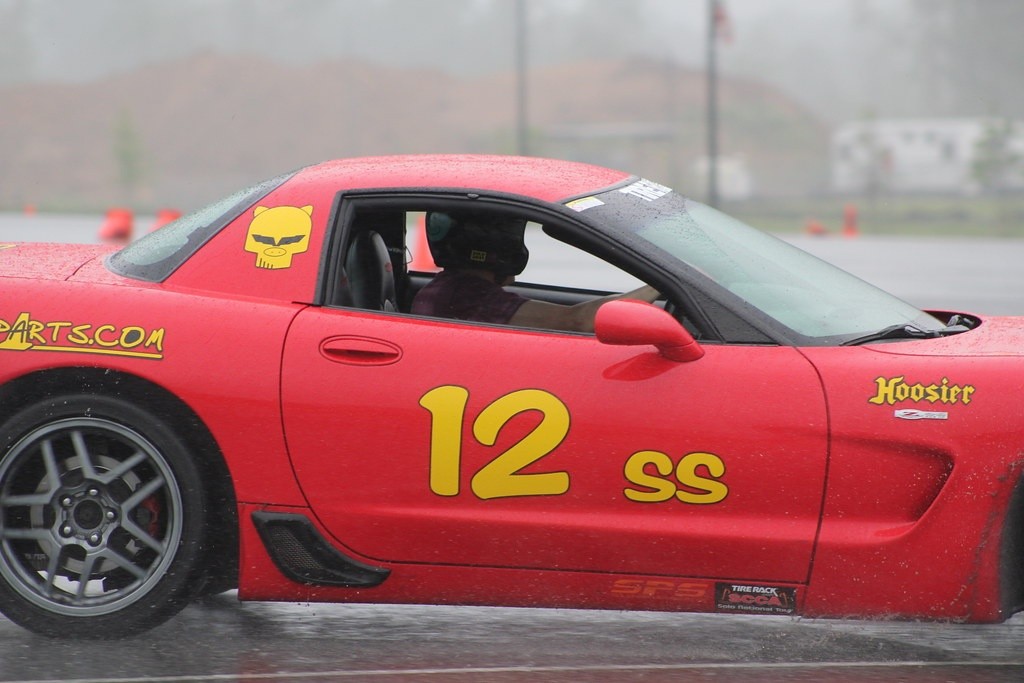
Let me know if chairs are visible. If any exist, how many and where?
[345,229,399,314]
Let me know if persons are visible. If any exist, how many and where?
[409,205,662,335]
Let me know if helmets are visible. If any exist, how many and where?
[426,205,530,276]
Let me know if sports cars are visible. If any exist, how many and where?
[1,155,1022,633]
